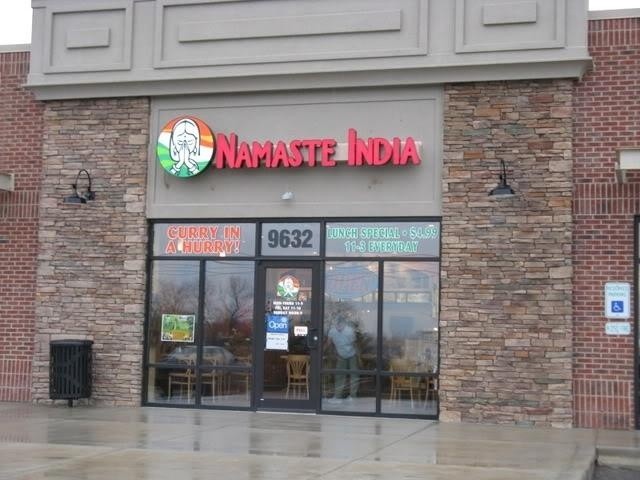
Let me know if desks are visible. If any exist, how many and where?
[319,368,438,377]
[148,363,249,397]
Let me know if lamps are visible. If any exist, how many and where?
[488,158,516,199]
[64,168,95,204]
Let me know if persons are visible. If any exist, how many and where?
[322,316,364,405]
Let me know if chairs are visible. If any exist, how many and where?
[386,357,435,411]
[279,354,310,400]
[167,355,251,401]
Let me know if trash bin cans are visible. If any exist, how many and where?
[49,339,93,406]
